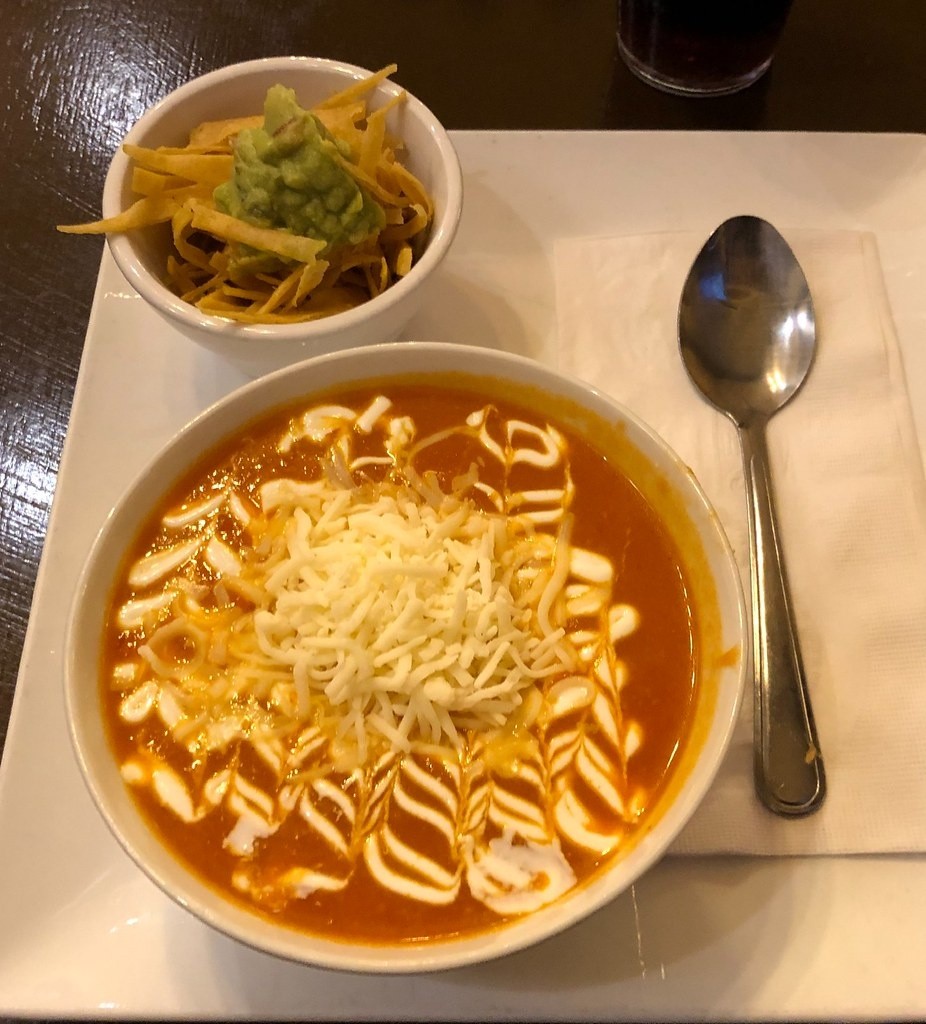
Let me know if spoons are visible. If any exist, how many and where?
[675,215,824,818]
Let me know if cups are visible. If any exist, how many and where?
[619,0,797,97]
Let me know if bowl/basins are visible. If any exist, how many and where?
[62,342,744,972]
[104,55,462,339]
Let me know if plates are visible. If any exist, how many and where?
[0,128,926,1022]
